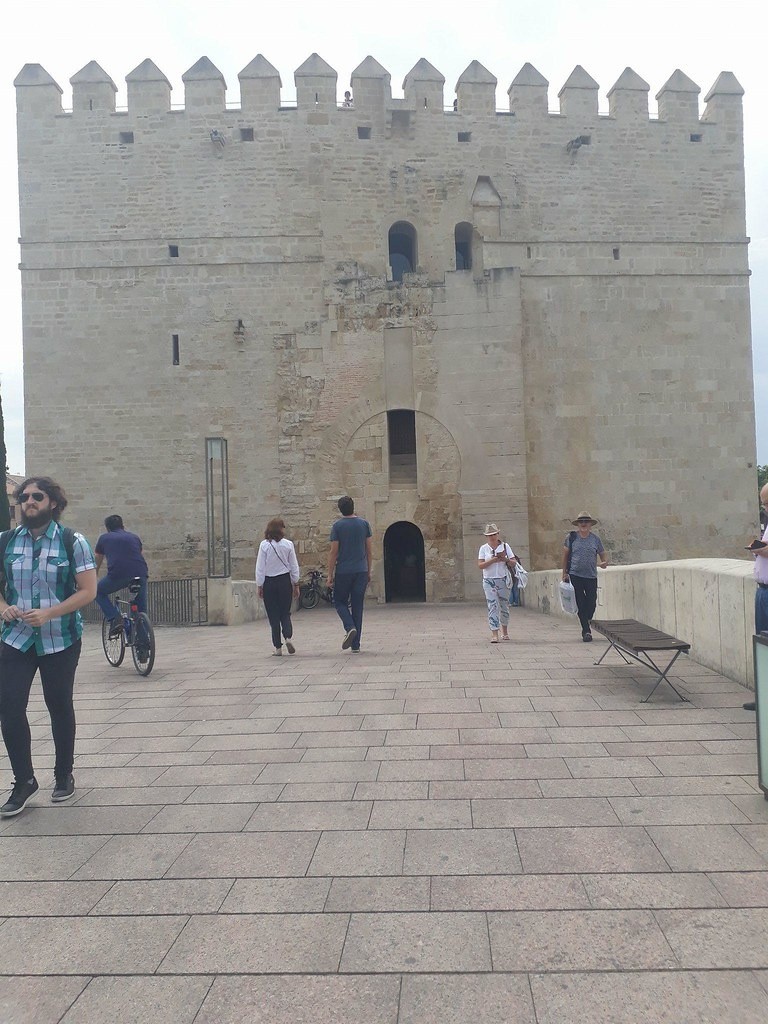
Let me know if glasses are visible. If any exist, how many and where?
[760,502,768,509]
[18,492,49,503]
[283,526,285,528]
[579,520,589,523]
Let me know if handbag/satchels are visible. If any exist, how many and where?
[559,574,578,615]
[504,543,516,583]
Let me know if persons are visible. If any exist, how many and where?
[742,482,768,711]
[477,523,517,644]
[0,476,99,817]
[327,495,372,653]
[562,510,608,642]
[93,515,152,663]
[255,517,300,657]
[508,553,522,607]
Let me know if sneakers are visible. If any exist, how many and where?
[0,776,40,816]
[51,774,75,801]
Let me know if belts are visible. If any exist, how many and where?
[757,583,768,590]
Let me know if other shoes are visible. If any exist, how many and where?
[138,654,149,663]
[109,618,124,636]
[285,640,295,654]
[502,635,509,640]
[508,602,518,606]
[491,637,499,642]
[342,627,357,650]
[584,632,592,641]
[272,651,282,656]
[352,648,360,652]
[742,702,756,711]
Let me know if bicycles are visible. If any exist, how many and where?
[300,564,352,609]
[101,577,155,676]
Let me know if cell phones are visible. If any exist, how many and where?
[497,550,507,558]
[745,540,767,550]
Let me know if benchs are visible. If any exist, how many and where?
[589,619,690,703]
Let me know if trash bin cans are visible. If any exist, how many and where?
[752,631,768,799]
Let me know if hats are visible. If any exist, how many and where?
[572,511,598,526]
[483,524,501,536]
